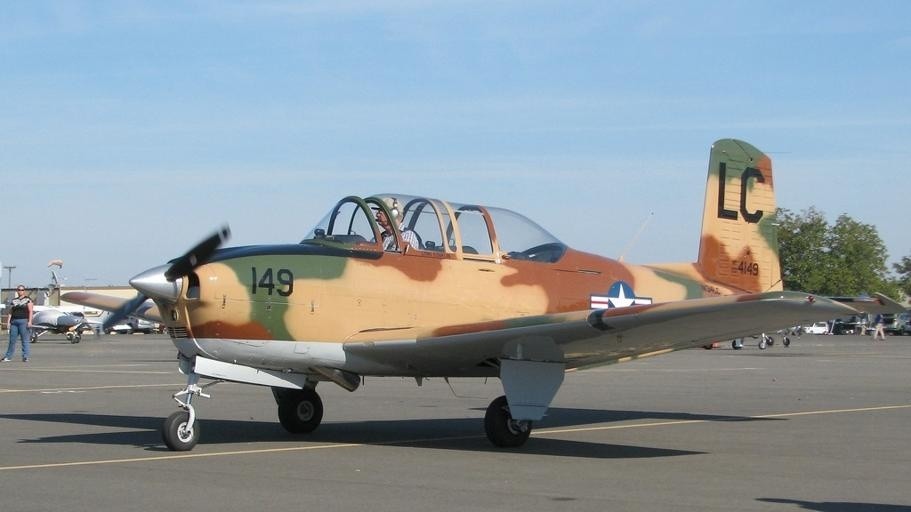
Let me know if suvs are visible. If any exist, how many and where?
[804,322,827,335]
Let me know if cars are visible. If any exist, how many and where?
[885,313,911,335]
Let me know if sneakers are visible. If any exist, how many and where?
[0,357,13,362]
[22,357,31,362]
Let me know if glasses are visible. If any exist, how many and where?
[17,288,25,292]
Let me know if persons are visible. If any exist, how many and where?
[1,285,37,363]
[371,197,423,252]
[873,302,887,340]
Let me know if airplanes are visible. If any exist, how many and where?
[61,138,910,450]
[0,302,105,344]
